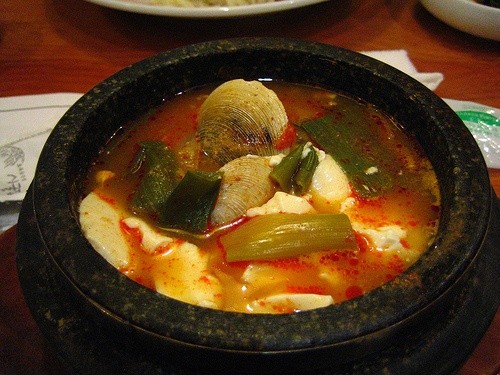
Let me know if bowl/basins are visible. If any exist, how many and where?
[423,0,500,41]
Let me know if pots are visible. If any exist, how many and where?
[15,42,500,375]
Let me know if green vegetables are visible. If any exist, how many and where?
[128,110,403,263]
